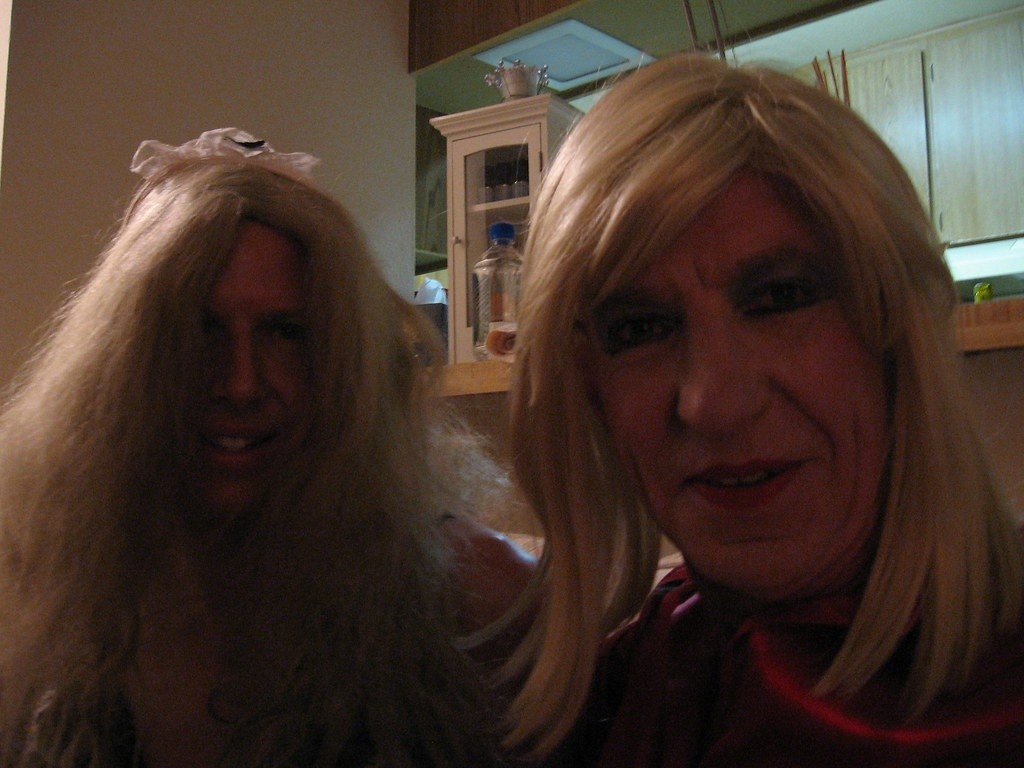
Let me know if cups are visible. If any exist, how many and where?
[476,181,528,204]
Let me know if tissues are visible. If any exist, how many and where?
[409,275,449,365]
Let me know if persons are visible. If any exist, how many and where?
[1,127,542,768]
[490,52,1024,768]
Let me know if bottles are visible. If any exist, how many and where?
[472,223,523,359]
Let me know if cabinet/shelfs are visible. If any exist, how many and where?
[407,0,1024,542]
